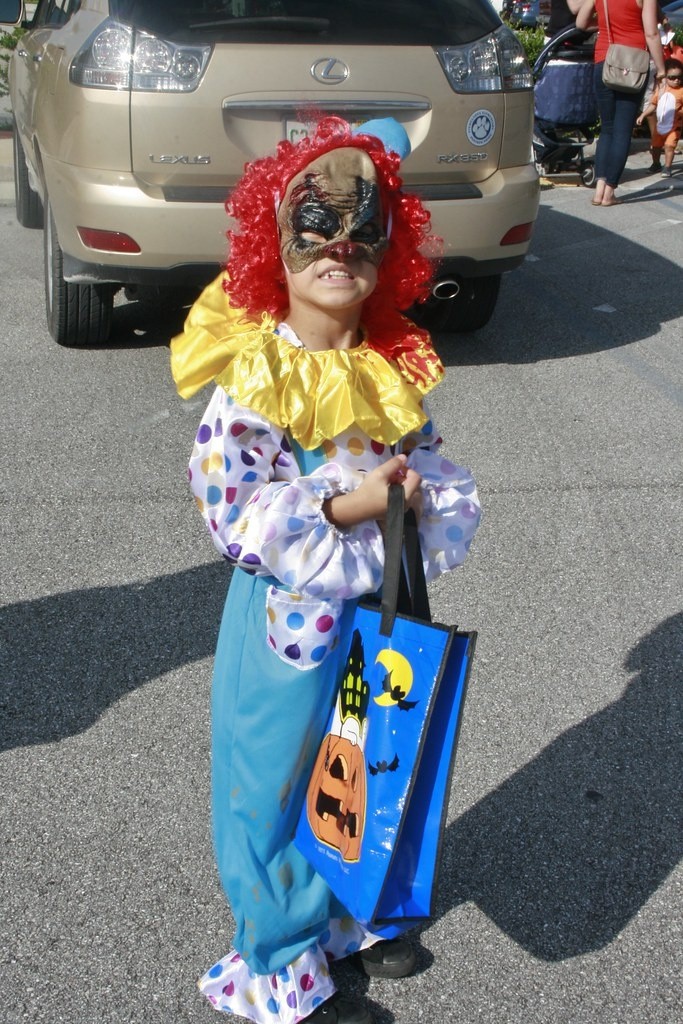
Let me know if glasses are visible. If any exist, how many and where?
[667,74,682,80]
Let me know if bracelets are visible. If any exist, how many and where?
[653,73,666,91]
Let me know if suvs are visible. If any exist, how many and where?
[0,0,542,350]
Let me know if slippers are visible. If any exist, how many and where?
[592,198,602,207]
[601,200,624,206]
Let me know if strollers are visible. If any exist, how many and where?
[524,21,601,188]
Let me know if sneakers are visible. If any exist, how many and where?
[349,926,418,980]
[292,992,373,1024]
[660,168,672,178]
[644,163,662,174]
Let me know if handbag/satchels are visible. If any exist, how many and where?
[602,42,652,91]
[291,479,480,941]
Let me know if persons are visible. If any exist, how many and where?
[544,0,683,207]
[170,113,483,1024]
